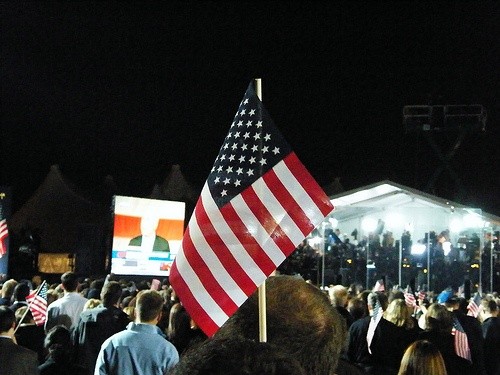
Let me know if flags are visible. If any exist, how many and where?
[169,80,334,339]
[467,292,481,318]
[458,284,464,298]
[404,285,416,306]
[370,279,384,293]
[450,312,472,361]
[150,276,160,291]
[25,280,47,325]
[0,216,9,276]
[416,291,426,306]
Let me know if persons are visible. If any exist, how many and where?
[127,216,170,253]
[0,306,38,375]
[0,218,500,375]
[94,289,179,375]
